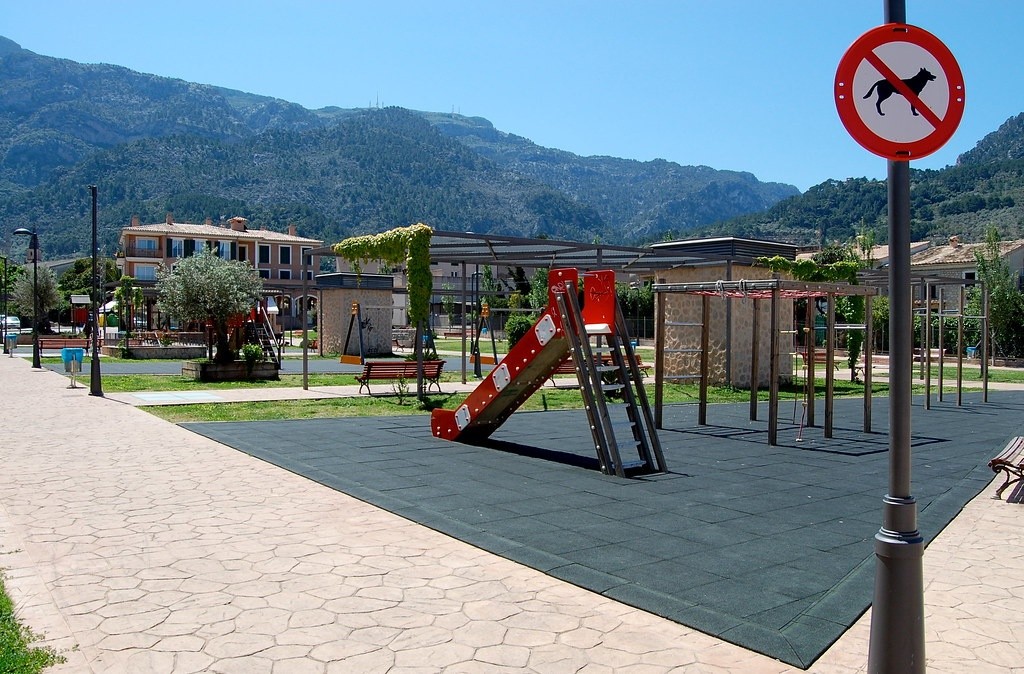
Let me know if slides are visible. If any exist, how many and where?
[429,302,568,443]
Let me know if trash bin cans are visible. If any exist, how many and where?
[61,348,84,373]
[6,335,17,349]
[482,328,487,335]
[967,347,976,359]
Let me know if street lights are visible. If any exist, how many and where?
[13,228,41,368]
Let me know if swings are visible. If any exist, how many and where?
[401,309,413,353]
[365,308,381,354]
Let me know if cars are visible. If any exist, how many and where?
[129,317,147,328]
[0,317,20,329]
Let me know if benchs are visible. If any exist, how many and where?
[592,355,651,378]
[97,339,143,350]
[309,340,318,353]
[543,359,590,387]
[444,333,468,339]
[987,436,1024,501]
[799,352,842,371]
[39,339,92,357]
[913,348,947,363]
[392,338,405,352]
[355,361,447,395]
